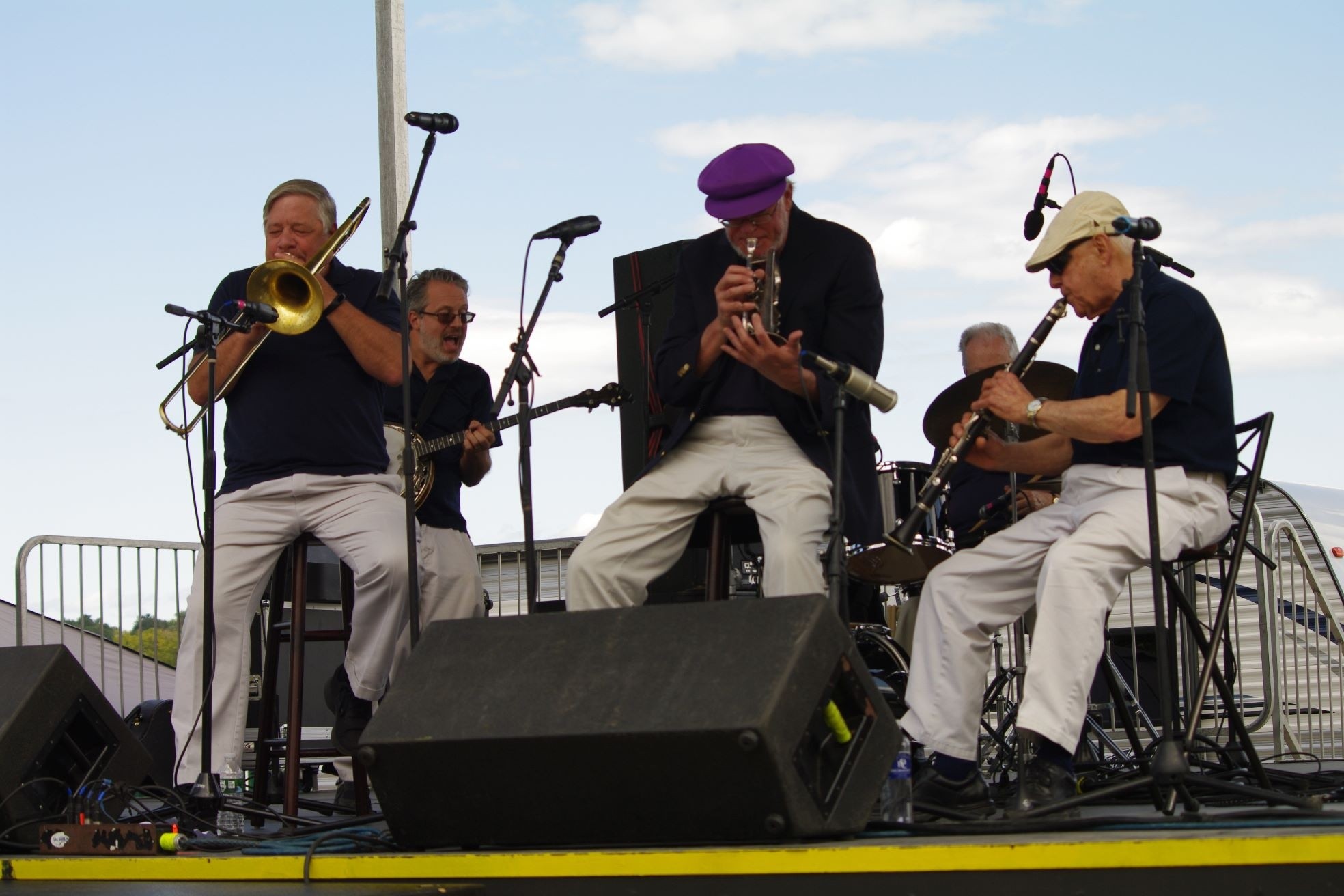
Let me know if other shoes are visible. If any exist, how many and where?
[333,777,357,813]
[136,782,195,819]
[324,664,373,752]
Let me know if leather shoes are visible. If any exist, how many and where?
[1005,759,1080,820]
[883,764,997,824]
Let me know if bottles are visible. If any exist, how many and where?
[214,755,245,837]
[878,718,916,826]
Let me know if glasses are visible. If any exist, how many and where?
[412,309,476,324]
[1045,236,1105,276]
[717,198,778,229]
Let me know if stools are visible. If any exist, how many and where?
[706,495,763,600]
[251,529,376,832]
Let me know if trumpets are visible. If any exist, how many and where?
[736,232,785,345]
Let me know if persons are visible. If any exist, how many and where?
[156,178,413,812]
[902,192,1237,819]
[377,267,491,708]
[564,142,882,615]
[892,323,1060,661]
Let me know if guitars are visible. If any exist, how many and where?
[382,380,625,517]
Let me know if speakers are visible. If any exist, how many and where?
[1086,621,1177,727]
[354,594,905,853]
[612,238,729,551]
[0,644,151,851]
[123,698,179,797]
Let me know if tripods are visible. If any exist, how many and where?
[117,305,385,846]
[993,238,1321,819]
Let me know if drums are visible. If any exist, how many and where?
[843,459,959,584]
[848,619,910,716]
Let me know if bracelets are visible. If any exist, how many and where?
[320,291,347,318]
[1050,493,1059,504]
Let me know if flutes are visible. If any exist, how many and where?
[883,303,1068,560]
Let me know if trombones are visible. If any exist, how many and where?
[154,194,376,434]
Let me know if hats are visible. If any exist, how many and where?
[1025,191,1130,274]
[698,143,795,220]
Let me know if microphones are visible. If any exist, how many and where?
[1024,156,1055,241]
[1113,216,1162,241]
[806,351,898,413]
[229,298,279,323]
[533,216,601,239]
[405,111,459,134]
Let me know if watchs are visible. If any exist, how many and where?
[1026,396,1049,429]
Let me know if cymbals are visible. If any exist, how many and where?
[922,359,1081,451]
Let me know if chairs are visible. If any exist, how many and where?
[1065,413,1274,804]
